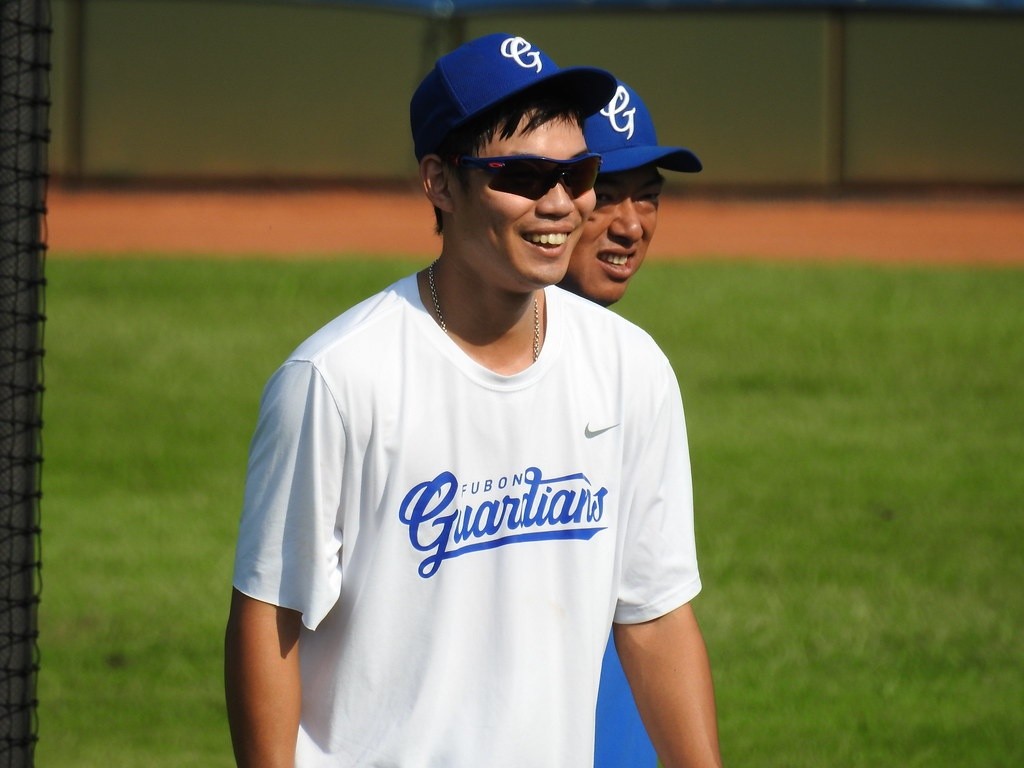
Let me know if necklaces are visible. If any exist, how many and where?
[429,258,538,365]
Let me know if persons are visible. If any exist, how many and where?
[570,70,666,767]
[224,31,726,767]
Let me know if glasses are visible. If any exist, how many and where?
[452,153,602,200]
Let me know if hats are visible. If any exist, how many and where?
[409,32,618,163]
[583,80,703,174]
